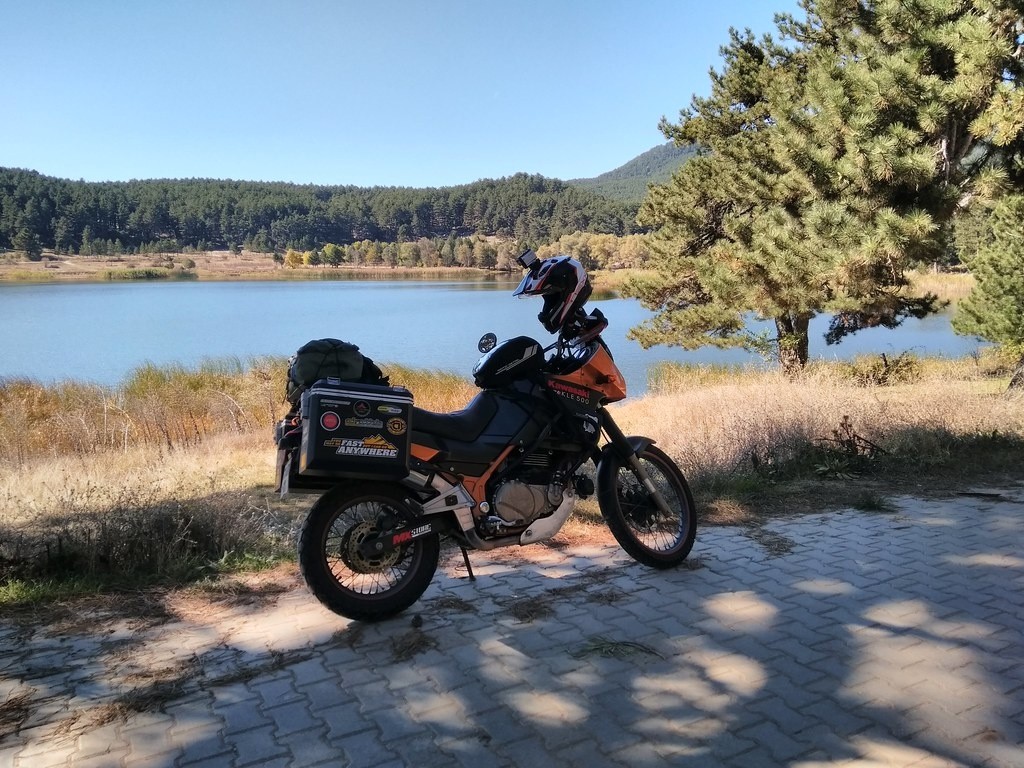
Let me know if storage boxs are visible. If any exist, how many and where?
[298,380,414,482]
[273,419,300,493]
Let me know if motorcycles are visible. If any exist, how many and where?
[274,295,699,624]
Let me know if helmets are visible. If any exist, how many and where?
[512,257,593,333]
